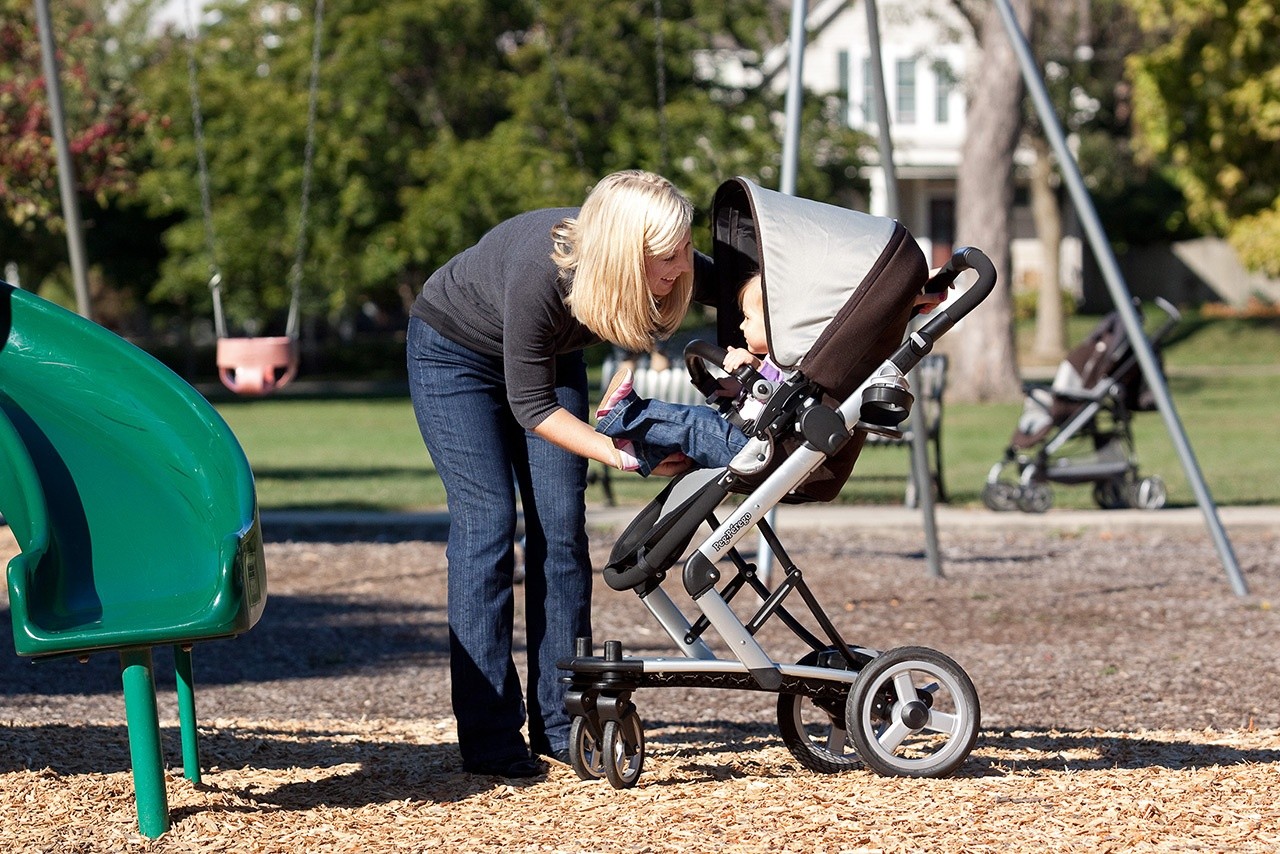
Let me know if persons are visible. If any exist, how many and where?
[595,274,786,478]
[407,170,955,779]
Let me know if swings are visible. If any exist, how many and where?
[169,0,324,407]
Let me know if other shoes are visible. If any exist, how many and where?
[537,750,594,770]
[595,368,634,421]
[605,435,640,472]
[477,760,545,779]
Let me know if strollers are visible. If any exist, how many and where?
[982,295,1185,514]
[554,177,999,792]
